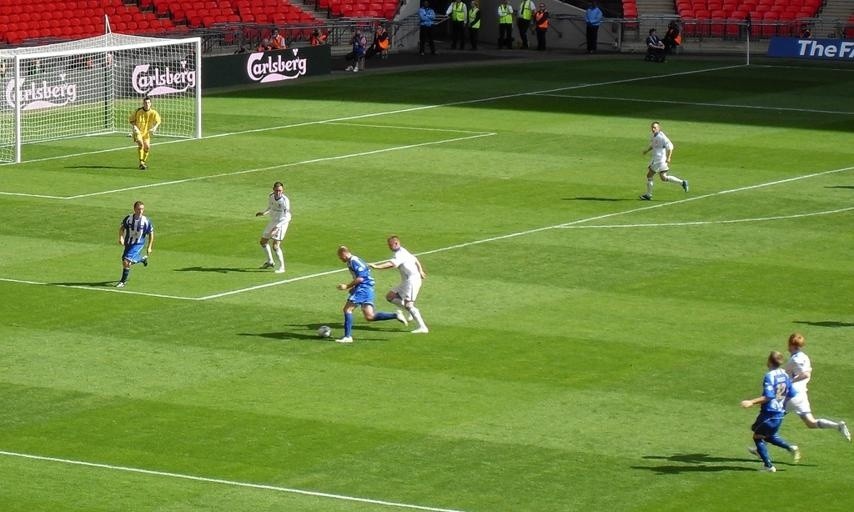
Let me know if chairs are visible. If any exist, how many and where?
[620,1,853,44]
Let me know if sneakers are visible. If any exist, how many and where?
[259,262,274,269]
[114,279,127,288]
[638,194,652,200]
[410,327,429,334]
[394,309,407,329]
[747,445,761,459]
[838,420,851,443]
[142,255,148,268]
[757,465,777,474]
[138,162,148,170]
[334,336,354,344]
[344,66,353,72]
[791,445,801,466]
[273,266,286,274]
[682,179,689,194]
[352,67,359,73]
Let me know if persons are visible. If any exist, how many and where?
[114,199,153,287]
[645,28,670,64]
[254,183,291,272]
[518,0,537,51]
[637,122,688,200]
[739,350,801,472]
[256,24,393,74]
[445,0,467,49]
[418,1,438,56]
[784,332,849,438]
[335,244,408,342]
[498,0,514,50]
[584,2,602,53]
[467,0,480,50]
[125,99,161,168]
[534,4,550,50]
[367,233,431,333]
[663,20,681,51]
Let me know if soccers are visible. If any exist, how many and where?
[318,326,331,337]
